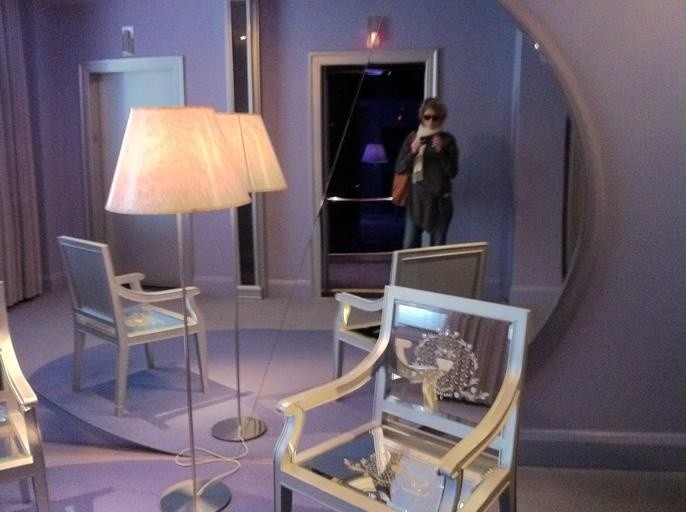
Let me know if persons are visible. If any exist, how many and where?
[395,96,459,250]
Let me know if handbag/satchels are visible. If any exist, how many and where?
[393,172,411,206]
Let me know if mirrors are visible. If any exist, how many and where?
[0,0,610,461]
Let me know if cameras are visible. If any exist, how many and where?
[420,136,431,144]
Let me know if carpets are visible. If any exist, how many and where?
[20,326,397,459]
[0,461,343,512]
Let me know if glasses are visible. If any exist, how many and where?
[423,115,439,121]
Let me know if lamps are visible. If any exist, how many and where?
[105,107,251,511]
[209,110,288,442]
[360,143,389,167]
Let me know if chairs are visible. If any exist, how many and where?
[57,235,209,417]
[330,240,489,401]
[0,281,55,512]
[273,283,531,512]
